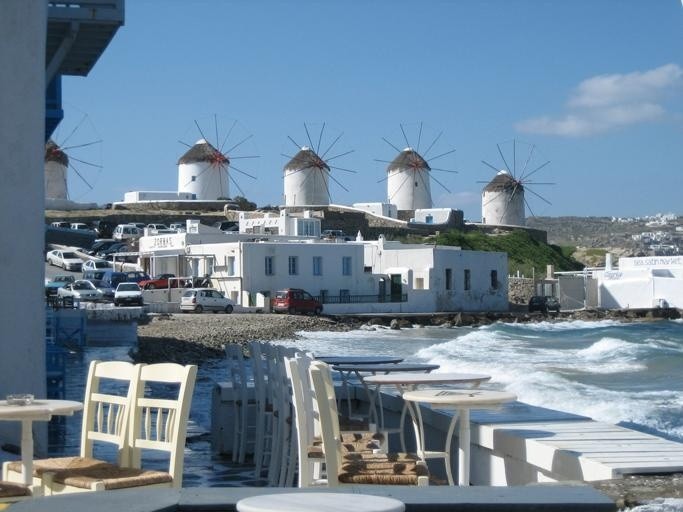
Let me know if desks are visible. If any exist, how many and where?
[235,492,406,512]
[331,364,517,486]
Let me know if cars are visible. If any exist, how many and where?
[272,288,323,315]
[45,239,185,307]
[180,288,235,314]
[322,227,355,240]
[529,296,561,314]
[51,221,185,239]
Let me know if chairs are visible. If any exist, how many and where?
[223,339,430,486]
[0,359,198,512]
